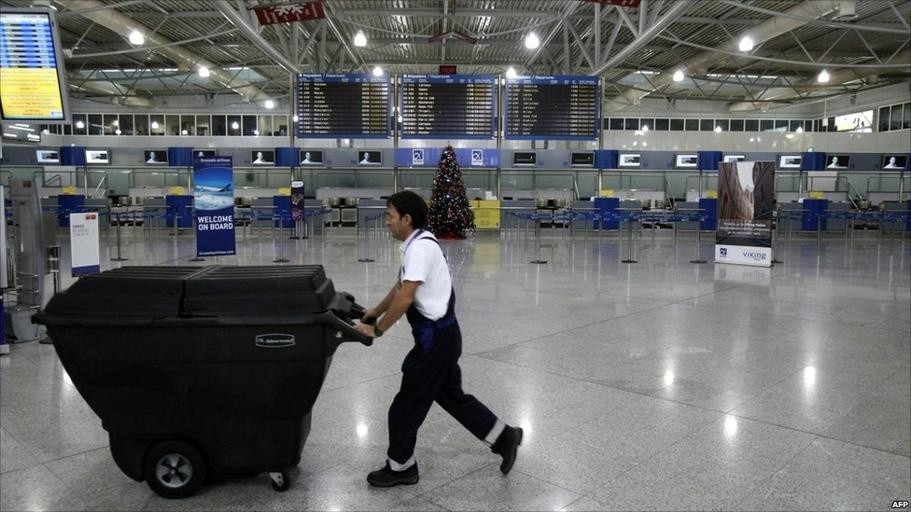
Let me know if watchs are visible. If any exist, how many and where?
[374,326,383,340]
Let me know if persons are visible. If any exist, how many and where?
[301,152,312,163]
[145,152,160,162]
[253,151,266,163]
[360,151,371,165]
[884,156,898,168]
[827,156,841,168]
[351,188,524,488]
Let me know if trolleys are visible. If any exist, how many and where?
[30,264,377,500]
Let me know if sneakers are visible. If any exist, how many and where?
[491,424,523,476]
[367,459,420,488]
